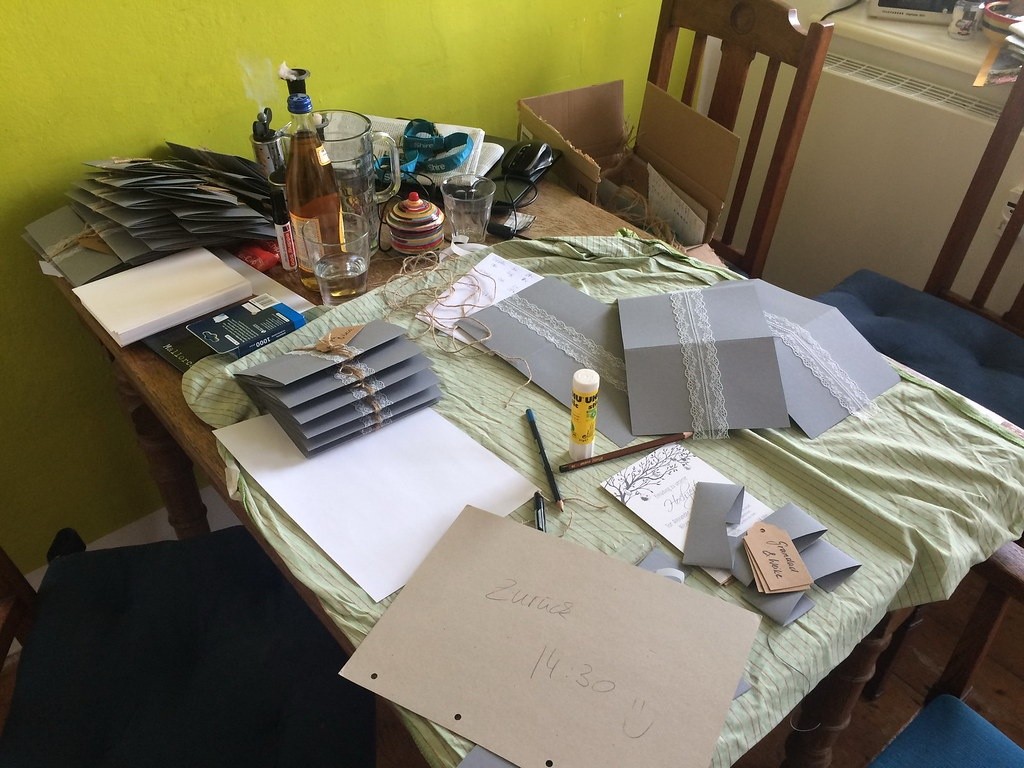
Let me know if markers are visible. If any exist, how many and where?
[269,189,298,272]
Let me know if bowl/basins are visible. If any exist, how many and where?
[983,0,1024,45]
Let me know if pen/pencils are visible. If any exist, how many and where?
[526,409,564,513]
[559,432,694,473]
[534,489,547,533]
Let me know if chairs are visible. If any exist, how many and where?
[643,0,834,281]
[1,525,372,767]
[808,70,1023,709]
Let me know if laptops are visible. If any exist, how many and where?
[478,136,562,208]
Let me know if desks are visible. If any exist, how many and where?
[42,184,1023,768]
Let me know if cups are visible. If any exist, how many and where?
[302,212,369,306]
[312,109,401,258]
[440,173,496,244]
[268,169,287,211]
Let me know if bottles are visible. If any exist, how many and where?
[284,92,346,292]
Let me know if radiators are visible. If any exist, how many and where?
[687,48,1024,321]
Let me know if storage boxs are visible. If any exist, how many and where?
[517,78,742,251]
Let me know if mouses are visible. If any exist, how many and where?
[500,140,553,175]
